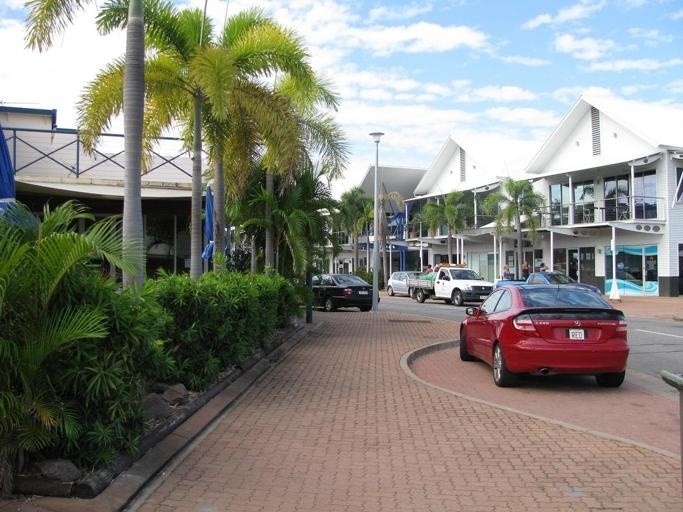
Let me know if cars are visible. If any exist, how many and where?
[302,272,380,314]
[457,284,627,388]
[386,269,421,299]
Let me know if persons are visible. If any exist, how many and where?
[504,265,512,280]
[522,259,530,280]
[536,262,550,272]
[424,264,433,275]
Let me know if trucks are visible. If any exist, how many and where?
[405,262,494,308]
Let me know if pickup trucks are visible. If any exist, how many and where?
[495,271,600,311]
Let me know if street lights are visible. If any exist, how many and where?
[367,129,387,313]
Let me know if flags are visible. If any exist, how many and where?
[205,186,213,242]
[0,127,16,220]
